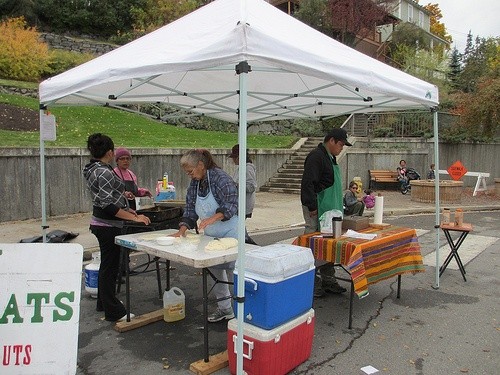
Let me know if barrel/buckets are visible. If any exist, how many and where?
[163,286,186,322]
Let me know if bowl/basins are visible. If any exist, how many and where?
[157,236,174,245]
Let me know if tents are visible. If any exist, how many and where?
[38,0,440,375]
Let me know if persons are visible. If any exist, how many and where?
[229,144,258,245]
[301,127,352,297]
[343,181,365,216]
[169,148,239,322]
[83,133,151,322]
[397,159,408,188]
[428,164,435,179]
[113,147,152,284]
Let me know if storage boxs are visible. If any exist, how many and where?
[228,309,314,375]
[231,242,316,330]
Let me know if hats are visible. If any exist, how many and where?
[329,128,353,147]
[114,148,130,161]
[229,144,248,158]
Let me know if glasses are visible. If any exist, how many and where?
[351,186,358,190]
[184,165,196,177]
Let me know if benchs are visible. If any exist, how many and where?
[368,169,398,190]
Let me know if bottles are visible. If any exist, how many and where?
[454,209,463,227]
[156,171,175,199]
[442,208,450,226]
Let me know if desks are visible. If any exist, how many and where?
[117,216,185,294]
[438,222,472,282]
[115,228,260,362]
[290,224,427,331]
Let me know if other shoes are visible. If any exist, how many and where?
[208,307,235,321]
[313,287,326,297]
[117,312,135,320]
[322,284,347,293]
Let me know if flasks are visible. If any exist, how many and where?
[332,216,342,238]
